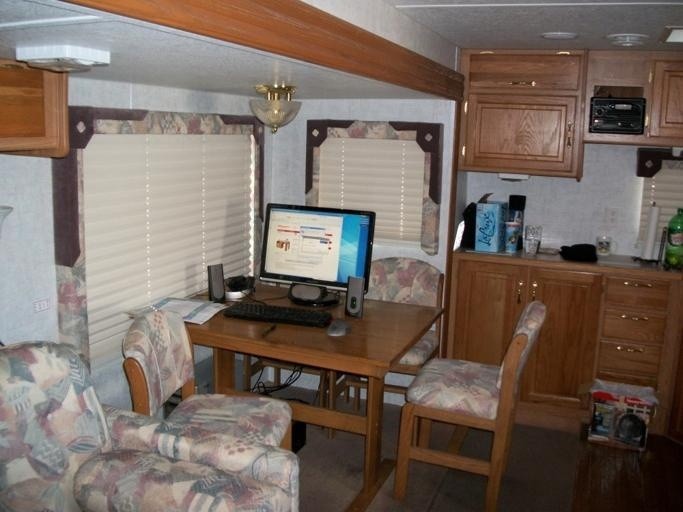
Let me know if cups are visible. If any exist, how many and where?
[523,224,541,257]
[595,236,618,257]
[502,220,521,255]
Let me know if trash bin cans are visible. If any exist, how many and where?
[283,398,309,453]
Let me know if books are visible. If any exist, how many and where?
[145,297,229,326]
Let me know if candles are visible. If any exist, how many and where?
[640,201,661,260]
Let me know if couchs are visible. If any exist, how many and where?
[0,338,303,511]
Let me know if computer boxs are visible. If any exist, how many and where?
[163,356,243,419]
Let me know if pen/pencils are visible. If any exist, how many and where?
[261,325,276,338]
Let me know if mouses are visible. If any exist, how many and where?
[327,319,351,337]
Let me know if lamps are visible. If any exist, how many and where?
[248,83,304,134]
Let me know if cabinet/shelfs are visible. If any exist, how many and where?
[648,60,683,139]
[452,257,605,411]
[457,47,584,183]
[592,275,683,441]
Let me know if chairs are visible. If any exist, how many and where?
[120,306,294,454]
[390,297,549,510]
[327,255,445,441]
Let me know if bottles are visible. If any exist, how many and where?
[663,208,682,269]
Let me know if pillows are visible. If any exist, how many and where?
[70,448,291,510]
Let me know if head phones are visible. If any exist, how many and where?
[224,275,255,299]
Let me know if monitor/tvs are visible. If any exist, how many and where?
[259,203,376,308]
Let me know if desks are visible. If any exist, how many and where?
[184,283,448,492]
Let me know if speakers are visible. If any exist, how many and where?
[207,264,225,303]
[345,276,365,318]
[288,283,325,302]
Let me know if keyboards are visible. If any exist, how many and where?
[225,302,332,328]
